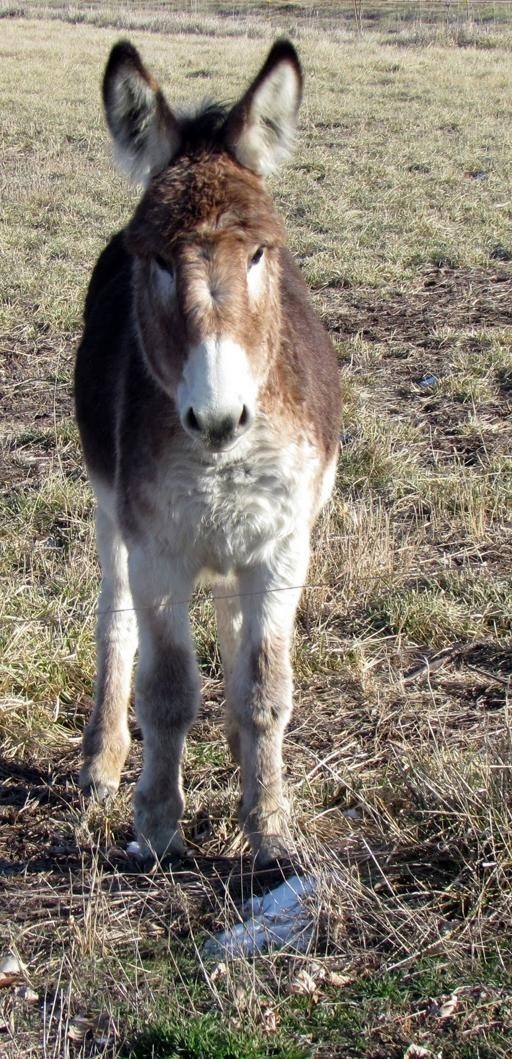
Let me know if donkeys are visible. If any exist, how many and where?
[74,35,344,884]
